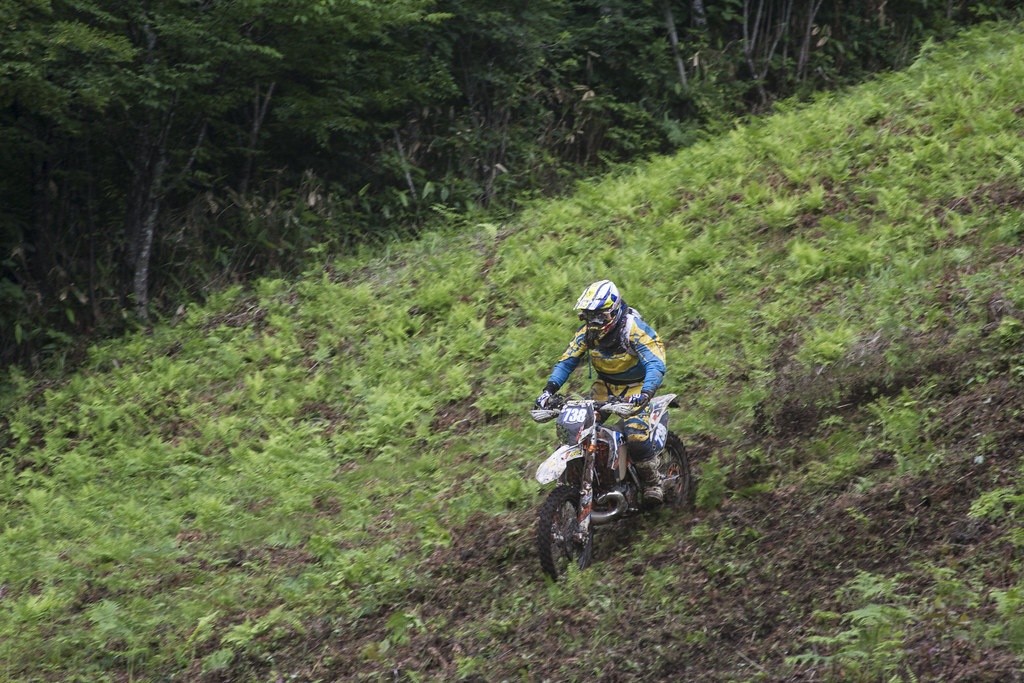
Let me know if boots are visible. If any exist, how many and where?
[630,454,663,503]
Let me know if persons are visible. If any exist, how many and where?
[533,280,668,509]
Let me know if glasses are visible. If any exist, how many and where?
[582,311,612,327]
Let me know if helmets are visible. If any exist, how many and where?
[573,280,622,341]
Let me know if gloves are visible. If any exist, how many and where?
[628,393,649,405]
[535,390,552,411]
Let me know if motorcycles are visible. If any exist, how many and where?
[528,393,692,585]
